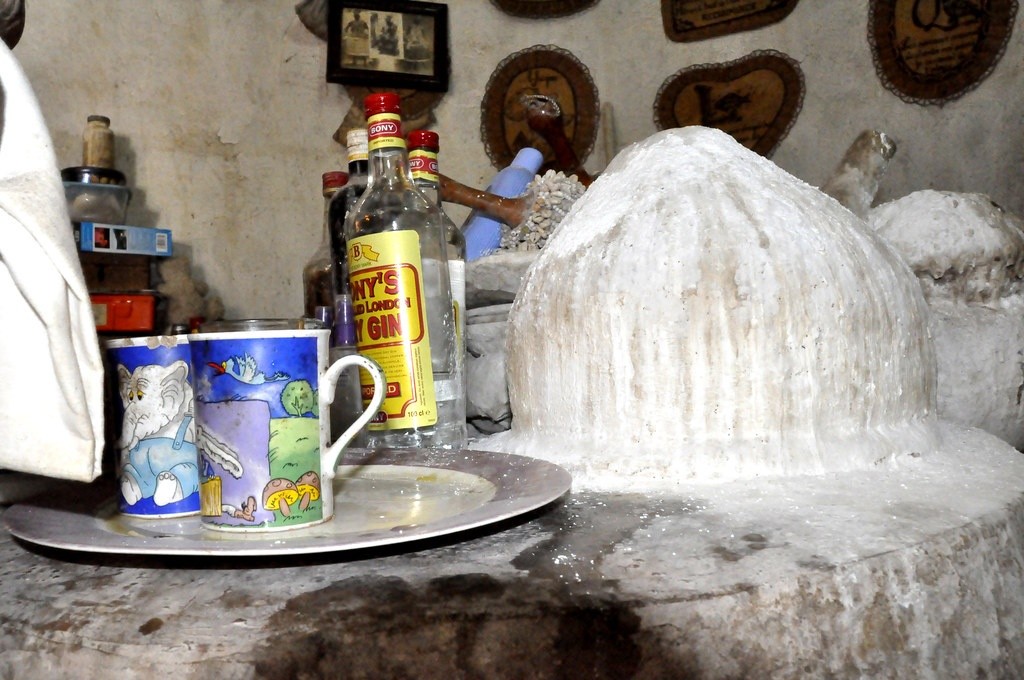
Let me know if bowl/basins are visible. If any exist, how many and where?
[62,182,129,224]
[62,167,126,205]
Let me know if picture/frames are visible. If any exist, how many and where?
[326,0,451,92]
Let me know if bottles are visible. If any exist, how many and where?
[82,115,113,168]
[303,91,468,450]
[460,148,542,262]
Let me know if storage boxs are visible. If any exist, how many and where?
[90,291,156,332]
[74,222,171,258]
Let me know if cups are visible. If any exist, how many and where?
[105,335,201,519]
[189,317,206,333]
[171,323,189,335]
[190,330,386,532]
[198,319,300,333]
